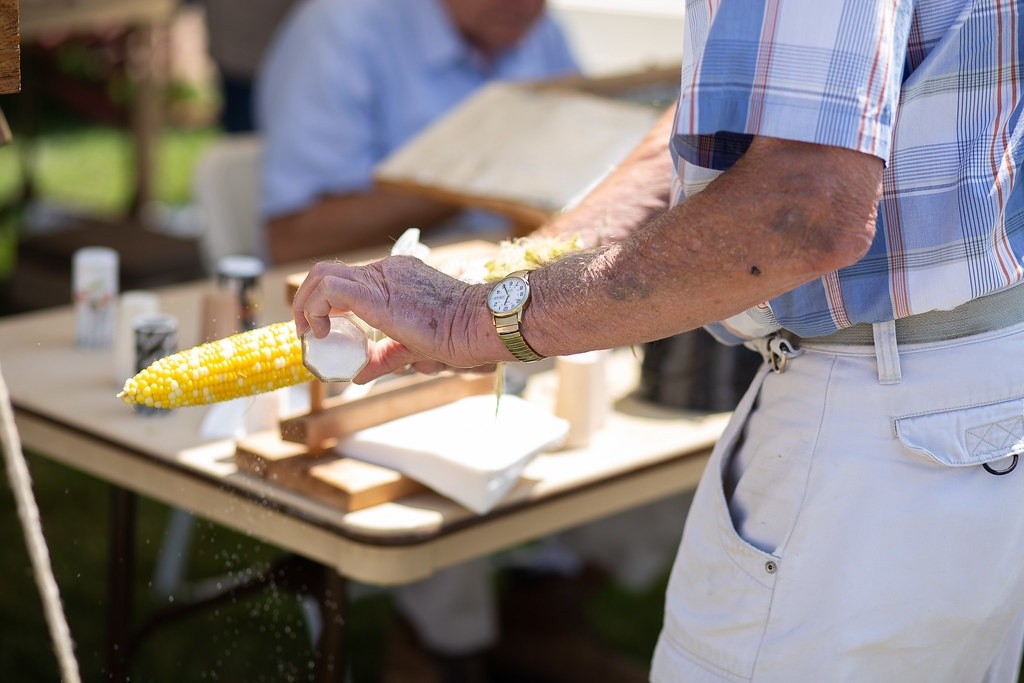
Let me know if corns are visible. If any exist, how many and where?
[116,313,318,409]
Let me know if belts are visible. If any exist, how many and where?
[780,281,1023,348]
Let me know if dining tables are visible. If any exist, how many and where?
[0,226,740,682]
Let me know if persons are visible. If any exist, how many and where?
[256,0,595,273]
[292,1,1024,683]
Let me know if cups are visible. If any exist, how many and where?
[209,256,268,342]
[73,245,121,347]
[133,322,176,417]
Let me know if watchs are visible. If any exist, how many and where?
[487,268,546,364]
